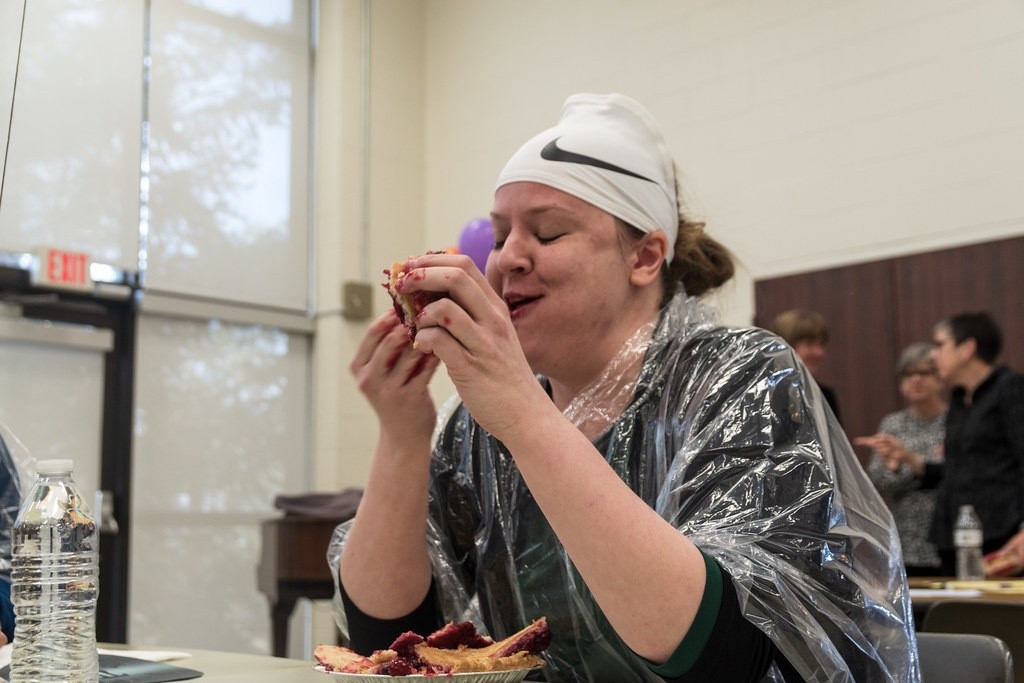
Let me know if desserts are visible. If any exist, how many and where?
[313,615,550,678]
[381,249,458,350]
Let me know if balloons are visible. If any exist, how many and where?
[459,217,497,276]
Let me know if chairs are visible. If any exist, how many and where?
[914,631,1014,682]
[919,599,1024,683]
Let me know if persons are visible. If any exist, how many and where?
[325,90,927,683]
[851,304,1024,582]
[766,297,844,431]
[865,340,955,579]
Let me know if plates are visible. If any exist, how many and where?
[312,657,547,683]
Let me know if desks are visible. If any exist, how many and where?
[902,577,1024,608]
[0,642,338,682]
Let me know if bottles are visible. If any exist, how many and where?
[9,458,99,683]
[953,506,985,582]
